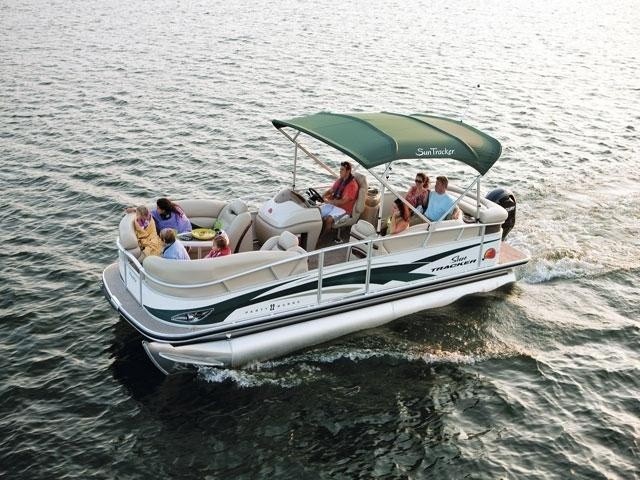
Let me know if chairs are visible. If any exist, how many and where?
[320,172,368,243]
[258,231,308,261]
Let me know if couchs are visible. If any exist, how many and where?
[120,199,252,264]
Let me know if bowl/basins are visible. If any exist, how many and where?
[191,228,216,241]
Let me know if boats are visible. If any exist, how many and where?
[102,111,530,376]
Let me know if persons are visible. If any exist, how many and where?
[151,199,193,233]
[204,236,231,258]
[127,206,151,229]
[160,228,191,259]
[405,173,460,227]
[381,198,409,236]
[317,162,359,244]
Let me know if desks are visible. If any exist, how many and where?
[164,233,223,260]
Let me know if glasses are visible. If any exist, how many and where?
[415,179,422,183]
